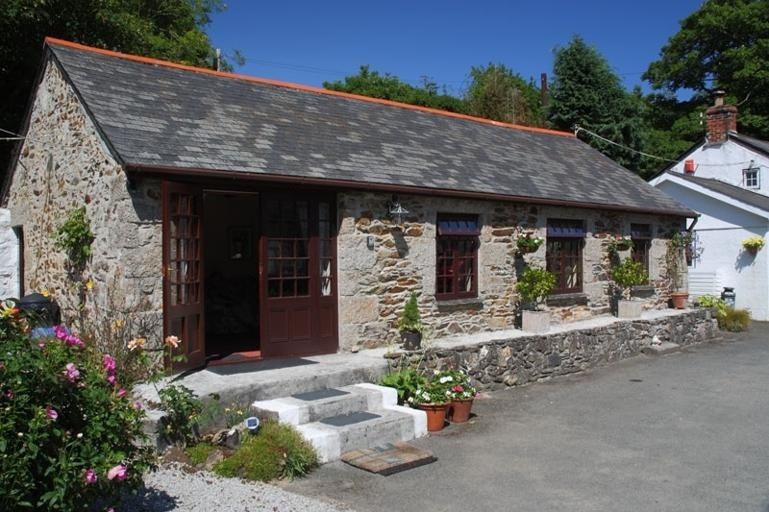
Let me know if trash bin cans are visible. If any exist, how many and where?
[721,287,736,309]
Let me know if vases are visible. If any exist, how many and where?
[671,291,689,310]
[749,247,758,254]
[419,399,472,430]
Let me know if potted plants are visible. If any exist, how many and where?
[518,267,557,333]
[672,230,692,247]
[516,235,543,253]
[399,291,423,350]
[614,260,645,319]
[611,238,634,251]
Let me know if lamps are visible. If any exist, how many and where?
[386,201,410,228]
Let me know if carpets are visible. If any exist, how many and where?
[343,441,436,475]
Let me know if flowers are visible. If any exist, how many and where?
[743,237,764,249]
[408,367,476,407]
